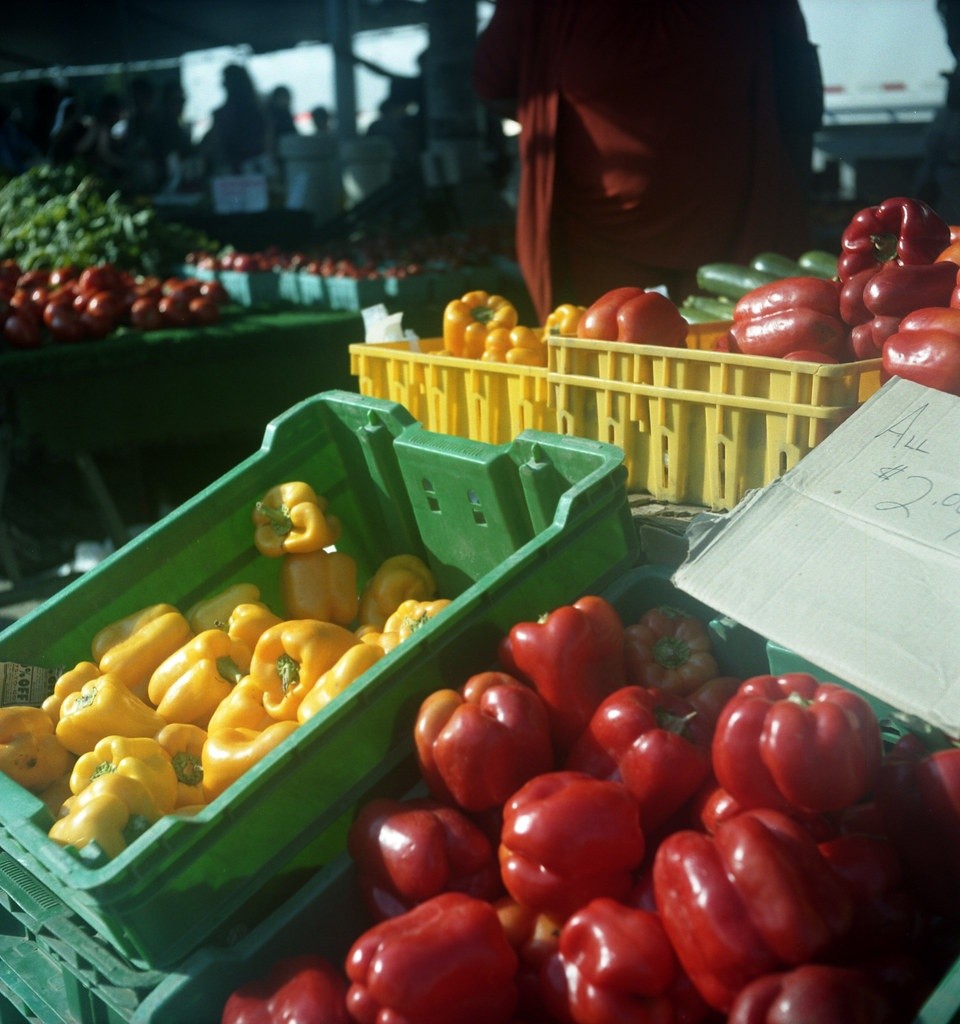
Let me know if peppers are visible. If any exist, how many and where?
[0,479,960,1024]
[435,199,960,393]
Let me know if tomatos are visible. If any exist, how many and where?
[3,247,419,333]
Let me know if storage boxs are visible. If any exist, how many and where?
[0,258,958,1024]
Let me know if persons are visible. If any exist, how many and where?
[466,1,825,328]
[0,44,430,218]
